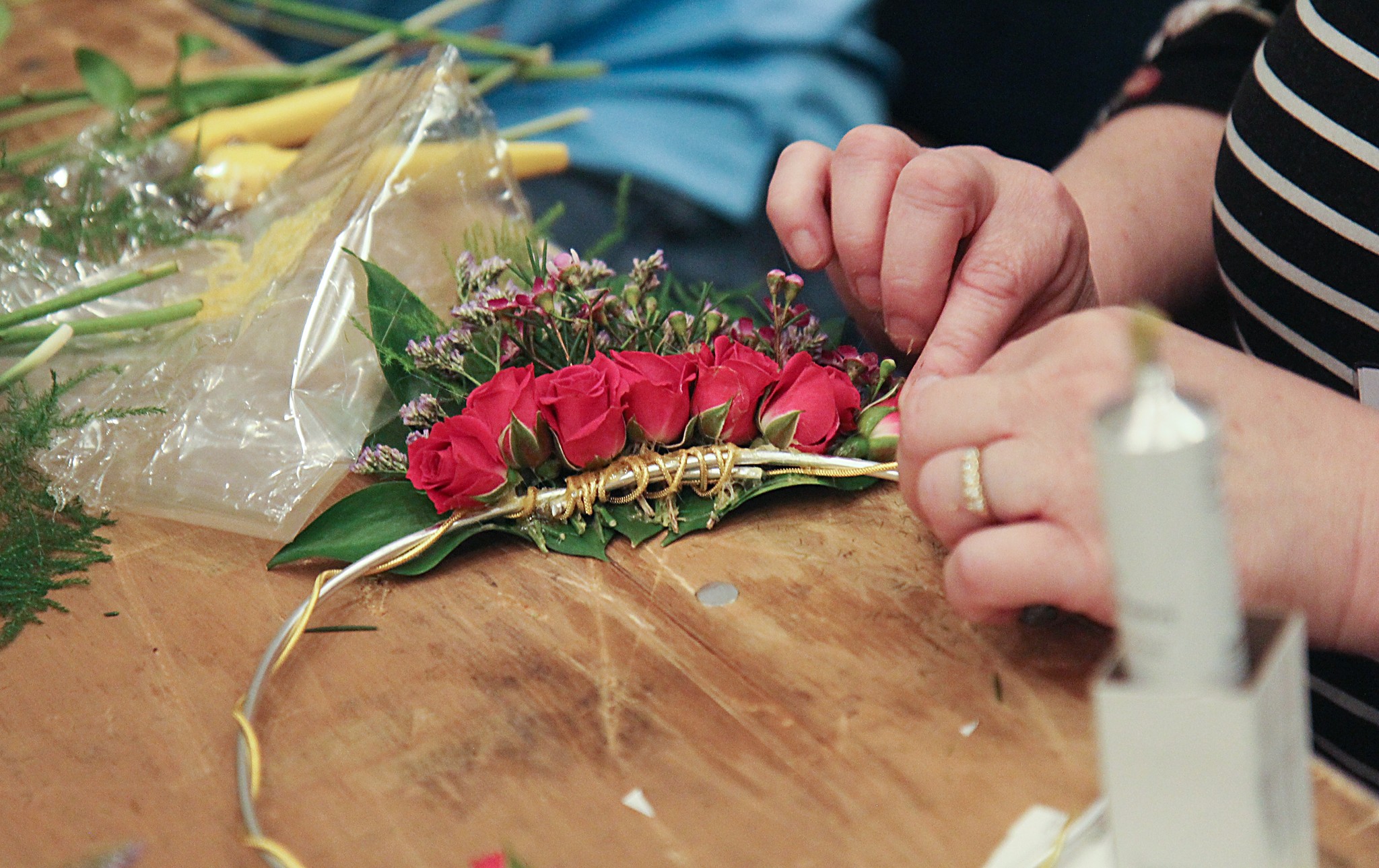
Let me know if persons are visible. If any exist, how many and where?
[202,0,884,365]
[766,0,1379,655]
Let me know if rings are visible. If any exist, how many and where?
[959,444,994,526]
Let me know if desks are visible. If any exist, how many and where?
[0,0,1379,868]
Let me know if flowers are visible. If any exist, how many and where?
[265,168,904,575]
